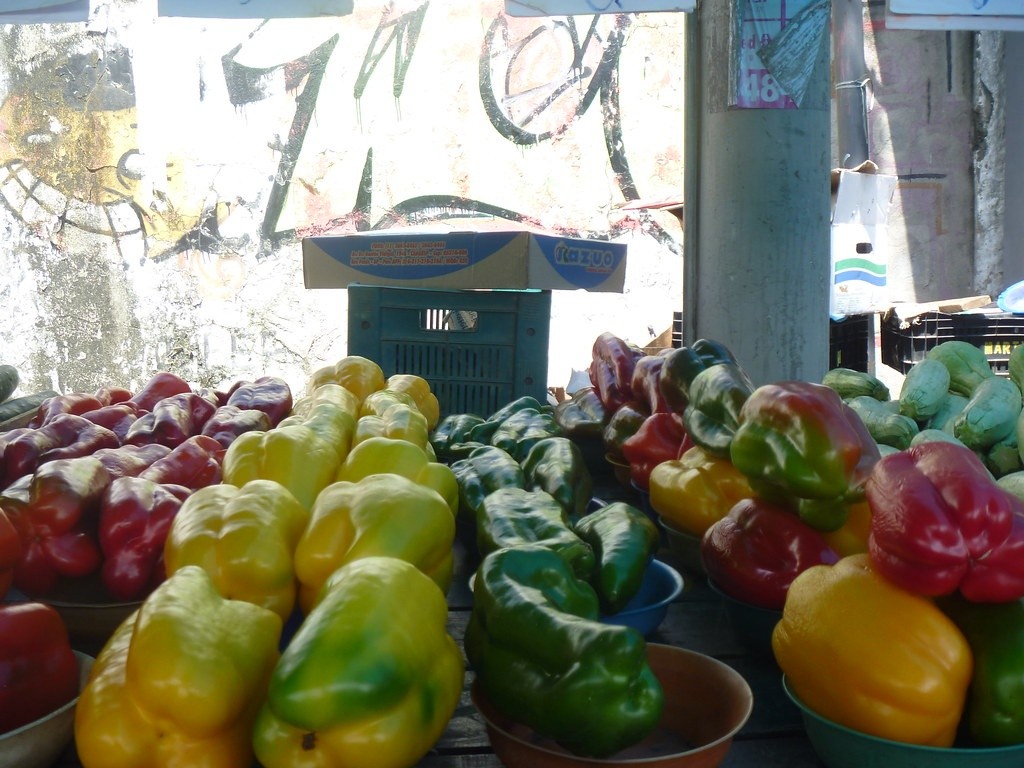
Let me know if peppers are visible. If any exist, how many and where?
[0,335,1023,768]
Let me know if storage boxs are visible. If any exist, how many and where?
[830,164,896,320]
[880,306,1024,378]
[644,312,672,354]
[300,231,629,294]
[830,316,868,374]
[347,284,553,431]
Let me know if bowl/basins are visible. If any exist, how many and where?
[8,449,1023,766]
